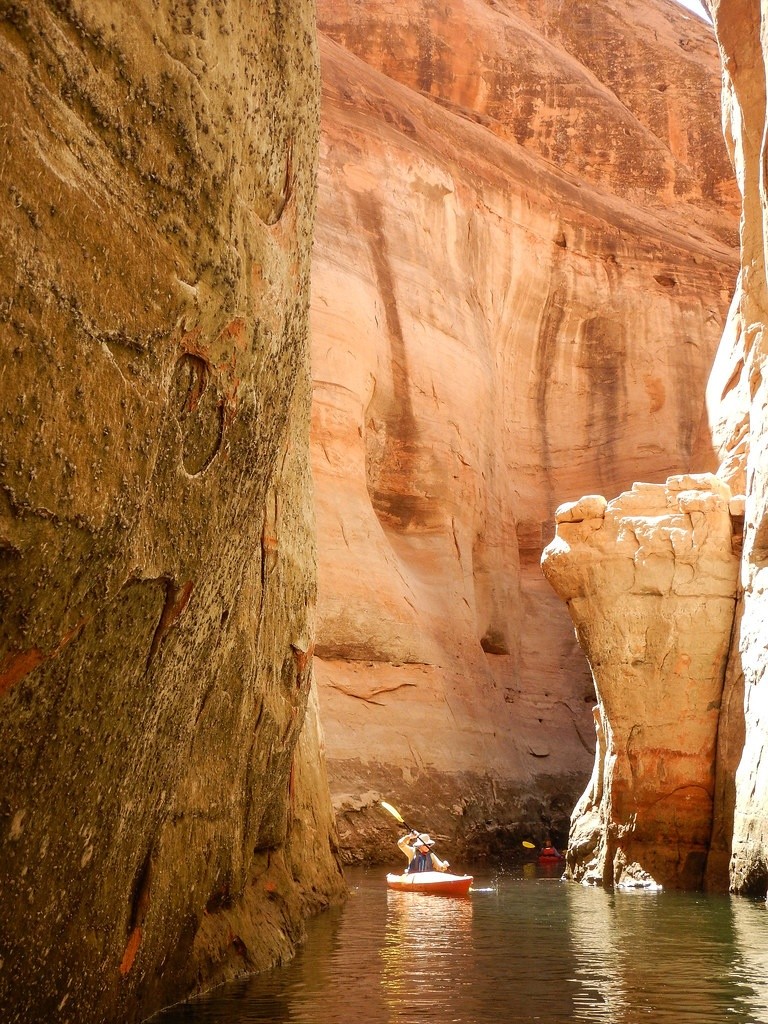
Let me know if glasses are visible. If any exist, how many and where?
[428,844,431,846]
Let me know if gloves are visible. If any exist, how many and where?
[442,861,450,868]
[410,831,419,839]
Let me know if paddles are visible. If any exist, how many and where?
[521,841,539,850]
[381,801,458,875]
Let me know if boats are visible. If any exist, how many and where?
[538,856,559,863]
[387,874,473,896]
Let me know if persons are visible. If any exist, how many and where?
[538,841,562,857]
[397,830,449,874]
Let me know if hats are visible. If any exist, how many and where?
[413,834,435,847]
